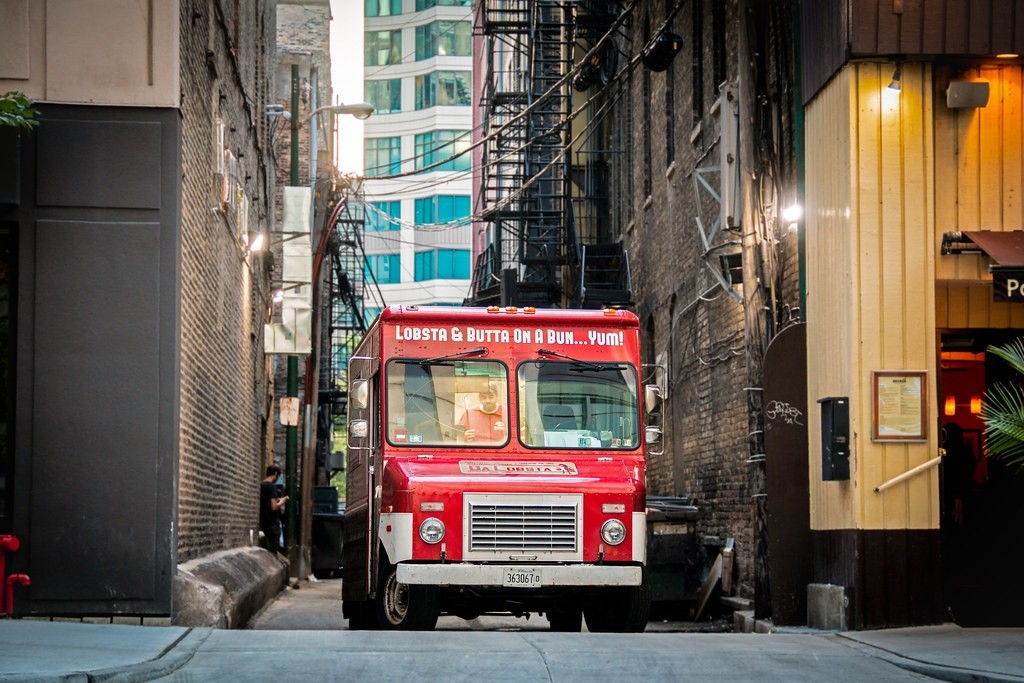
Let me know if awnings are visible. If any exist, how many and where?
[960,229,1024,304]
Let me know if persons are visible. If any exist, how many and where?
[456,385,508,443]
[260,465,289,554]
[939,421,983,624]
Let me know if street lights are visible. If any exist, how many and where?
[285,102,374,580]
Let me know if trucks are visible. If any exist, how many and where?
[342,305,653,632]
